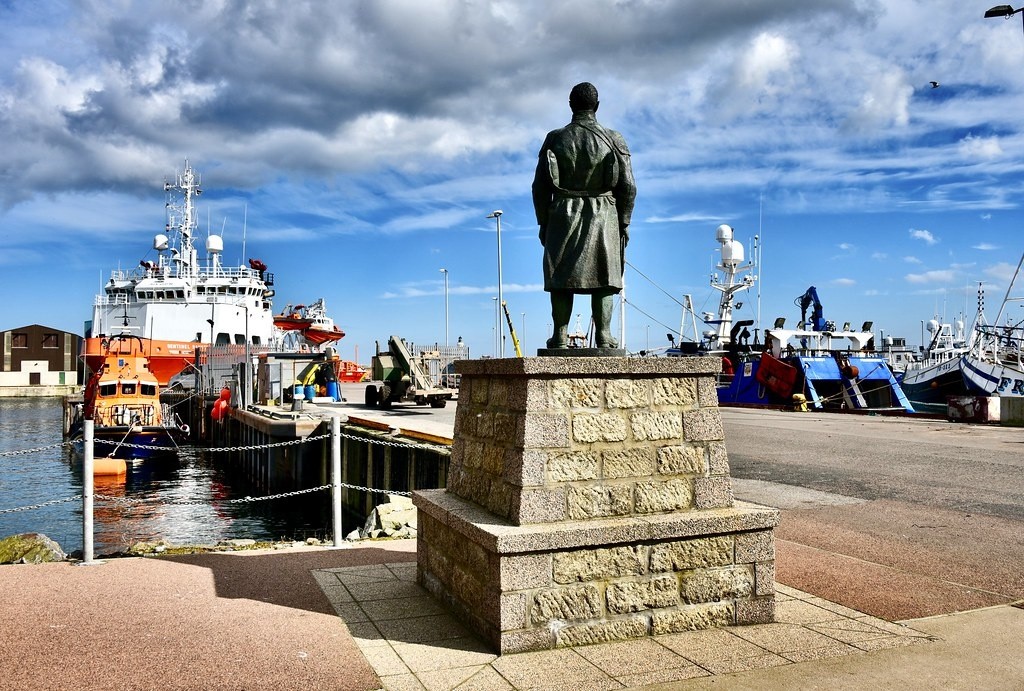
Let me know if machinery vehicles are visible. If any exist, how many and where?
[365,335,452,411]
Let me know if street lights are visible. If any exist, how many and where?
[487,211,506,360]
[440,269,450,386]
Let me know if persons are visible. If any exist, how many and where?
[135,416,141,426]
[531,82,636,348]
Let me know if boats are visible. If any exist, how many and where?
[78,156,342,403]
[664,220,1023,418]
[66,335,191,457]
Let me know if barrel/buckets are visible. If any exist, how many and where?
[293,384,304,394]
[304,384,316,400]
[326,381,340,401]
